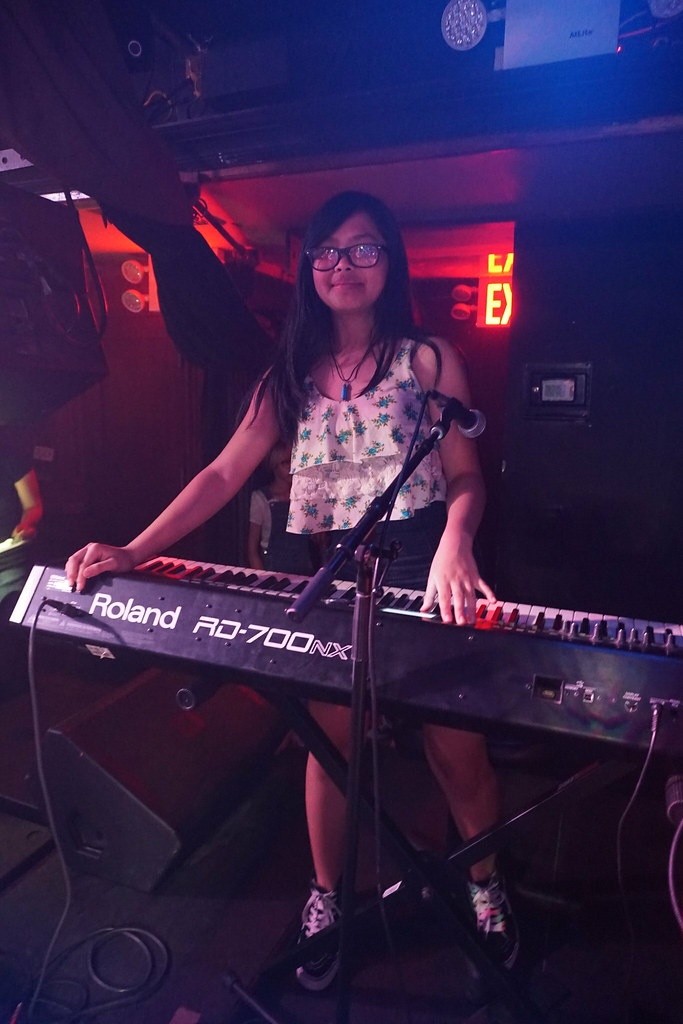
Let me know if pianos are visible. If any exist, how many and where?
[6,549,683,761]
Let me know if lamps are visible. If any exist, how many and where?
[441,0,683,71]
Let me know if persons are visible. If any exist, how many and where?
[66,190,518,990]
[0,422,44,546]
[247,439,328,574]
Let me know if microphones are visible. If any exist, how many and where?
[428,388,486,438]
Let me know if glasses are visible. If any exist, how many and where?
[304,243,389,272]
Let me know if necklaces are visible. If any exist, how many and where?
[325,339,375,399]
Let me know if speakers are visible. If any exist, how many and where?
[0,181,113,427]
[21,662,287,894]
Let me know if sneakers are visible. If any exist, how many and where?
[464,869,520,986]
[296,869,362,991]
